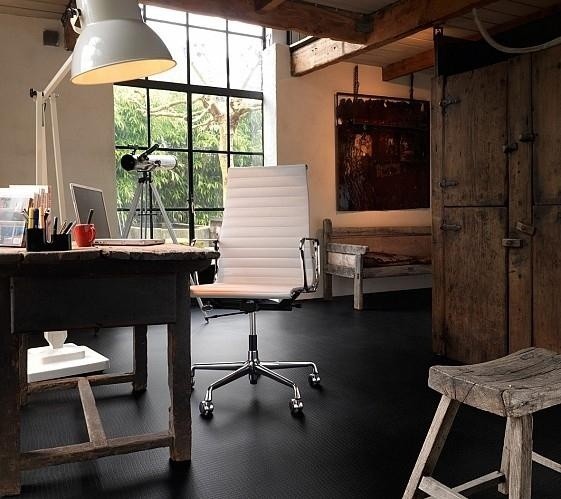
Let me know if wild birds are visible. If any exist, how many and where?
[70,182,167,246]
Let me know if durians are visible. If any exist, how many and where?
[121,144,178,173]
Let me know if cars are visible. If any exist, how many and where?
[74,223,97,248]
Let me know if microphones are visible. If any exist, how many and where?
[402,346,561,499]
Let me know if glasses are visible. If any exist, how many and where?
[29,1,178,233]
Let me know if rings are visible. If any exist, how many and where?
[88,209,94,224]
[20,205,77,235]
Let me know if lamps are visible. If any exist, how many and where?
[190,162,322,420]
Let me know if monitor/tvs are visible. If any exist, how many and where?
[0,185,52,247]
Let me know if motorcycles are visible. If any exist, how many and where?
[120,176,210,323]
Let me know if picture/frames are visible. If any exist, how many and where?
[0,243,221,496]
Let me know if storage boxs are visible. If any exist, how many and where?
[322,218,432,310]
